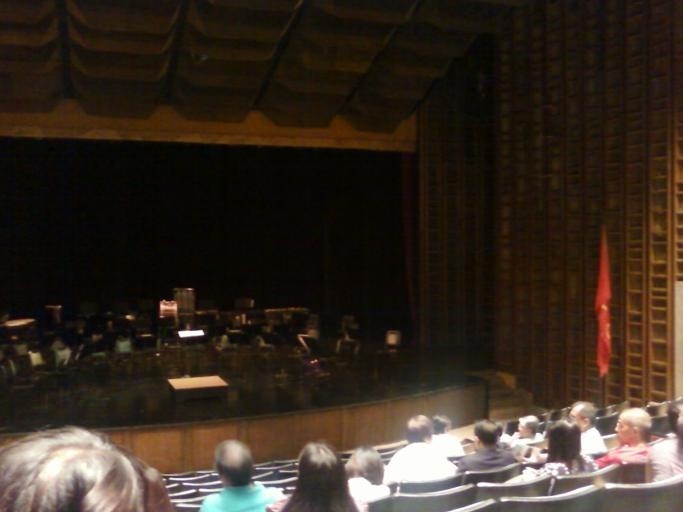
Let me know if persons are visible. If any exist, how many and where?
[387,402,682,483]
[1,300,365,382]
[1,426,390,512]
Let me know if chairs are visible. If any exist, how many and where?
[151,393,683,511]
[0,300,405,380]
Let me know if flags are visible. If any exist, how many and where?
[593,222,614,377]
